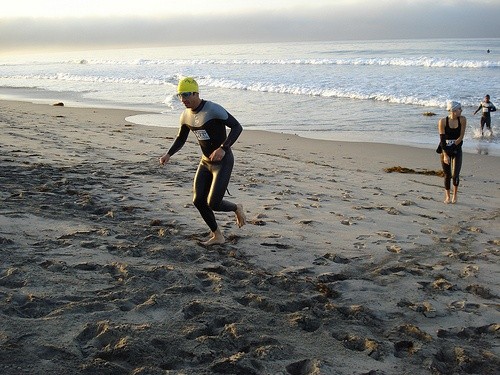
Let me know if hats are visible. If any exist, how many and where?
[446,101,461,111]
[176,77,200,94]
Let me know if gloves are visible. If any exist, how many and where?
[443,143,457,157]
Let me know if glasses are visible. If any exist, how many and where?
[176,92,194,98]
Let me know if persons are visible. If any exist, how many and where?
[473,94,497,137]
[436,101,466,204]
[160,77,246,246]
